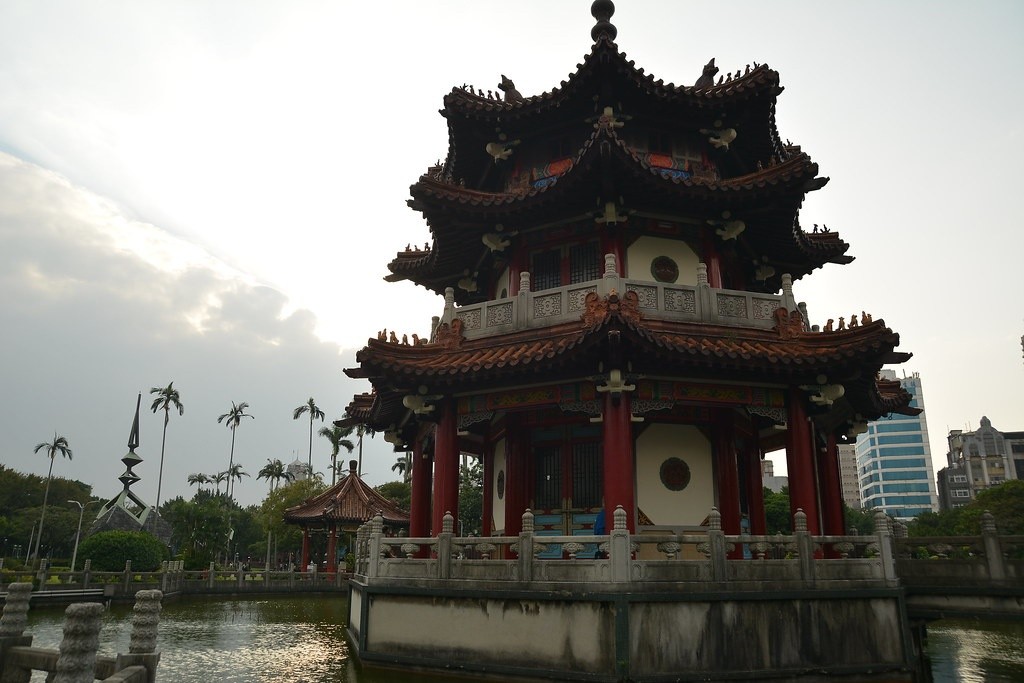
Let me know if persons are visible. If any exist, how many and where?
[243,563,246,579]
[466,533,477,558]
[203,566,207,580]
[593,496,605,559]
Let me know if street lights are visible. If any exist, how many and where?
[65,500,100,583]
